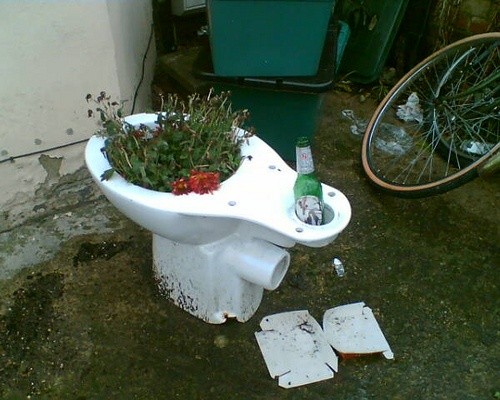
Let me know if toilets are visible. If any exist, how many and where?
[83,111,353,325]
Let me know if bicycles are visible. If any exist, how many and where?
[361,31,499,200]
[421,45,500,171]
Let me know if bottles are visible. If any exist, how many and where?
[292,136,326,226]
